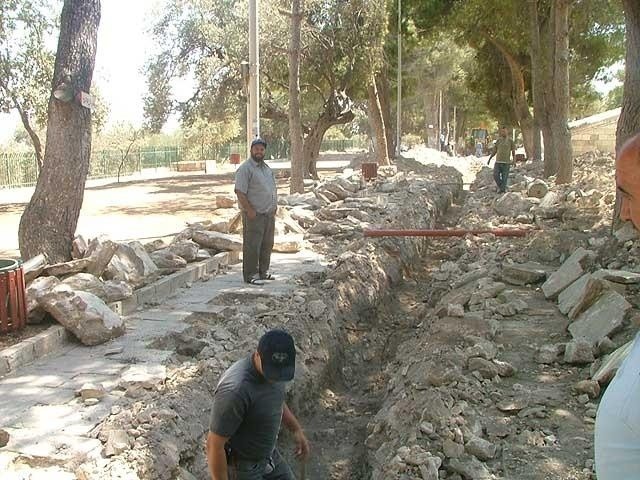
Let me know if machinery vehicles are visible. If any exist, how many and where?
[466,127,492,156]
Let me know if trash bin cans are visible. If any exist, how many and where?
[230,154,240,164]
[362,163,377,179]
[0,257,28,331]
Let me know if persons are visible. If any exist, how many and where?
[475,139,484,158]
[487,125,517,194]
[204,329,311,480]
[594,131,640,480]
[234,138,278,286]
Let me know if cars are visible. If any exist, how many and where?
[439,134,446,144]
[394,141,411,154]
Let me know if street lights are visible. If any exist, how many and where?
[241,58,251,161]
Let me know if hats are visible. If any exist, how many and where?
[257,331,296,382]
[251,138,267,146]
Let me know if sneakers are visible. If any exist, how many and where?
[266,273,276,281]
[250,276,264,285]
